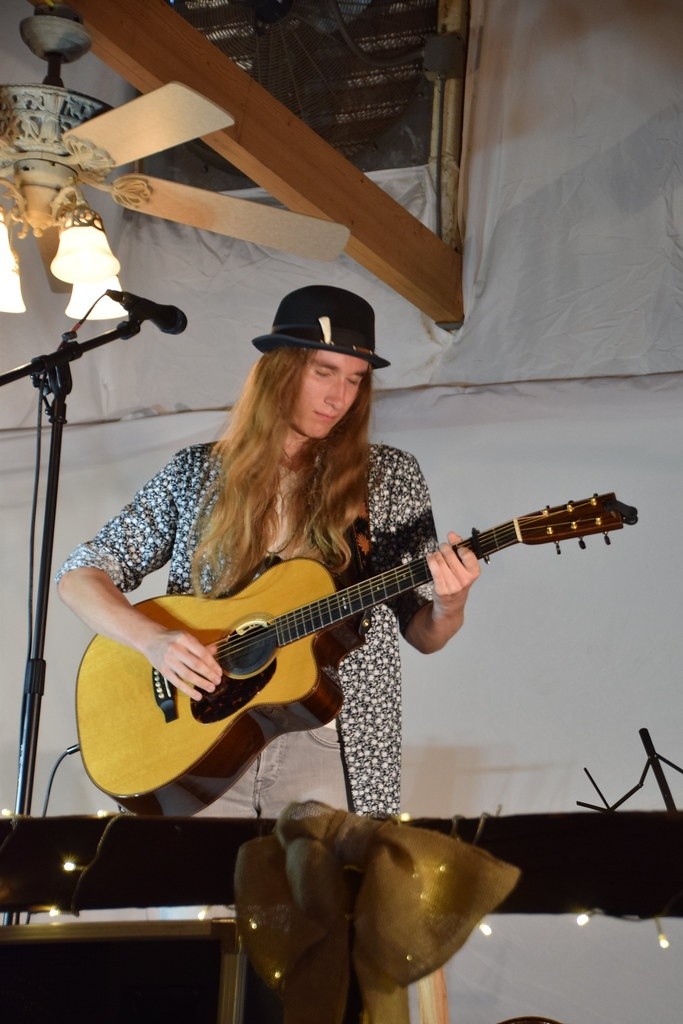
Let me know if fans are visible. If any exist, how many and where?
[0,5,350,293]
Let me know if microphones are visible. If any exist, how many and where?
[106,289,187,335]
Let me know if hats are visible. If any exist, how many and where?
[251,285,391,369]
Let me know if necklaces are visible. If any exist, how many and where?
[267,520,302,554]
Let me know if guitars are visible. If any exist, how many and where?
[74,488,642,816]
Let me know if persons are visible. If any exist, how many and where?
[57,286,480,819]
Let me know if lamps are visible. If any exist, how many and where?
[0,158,129,320]
